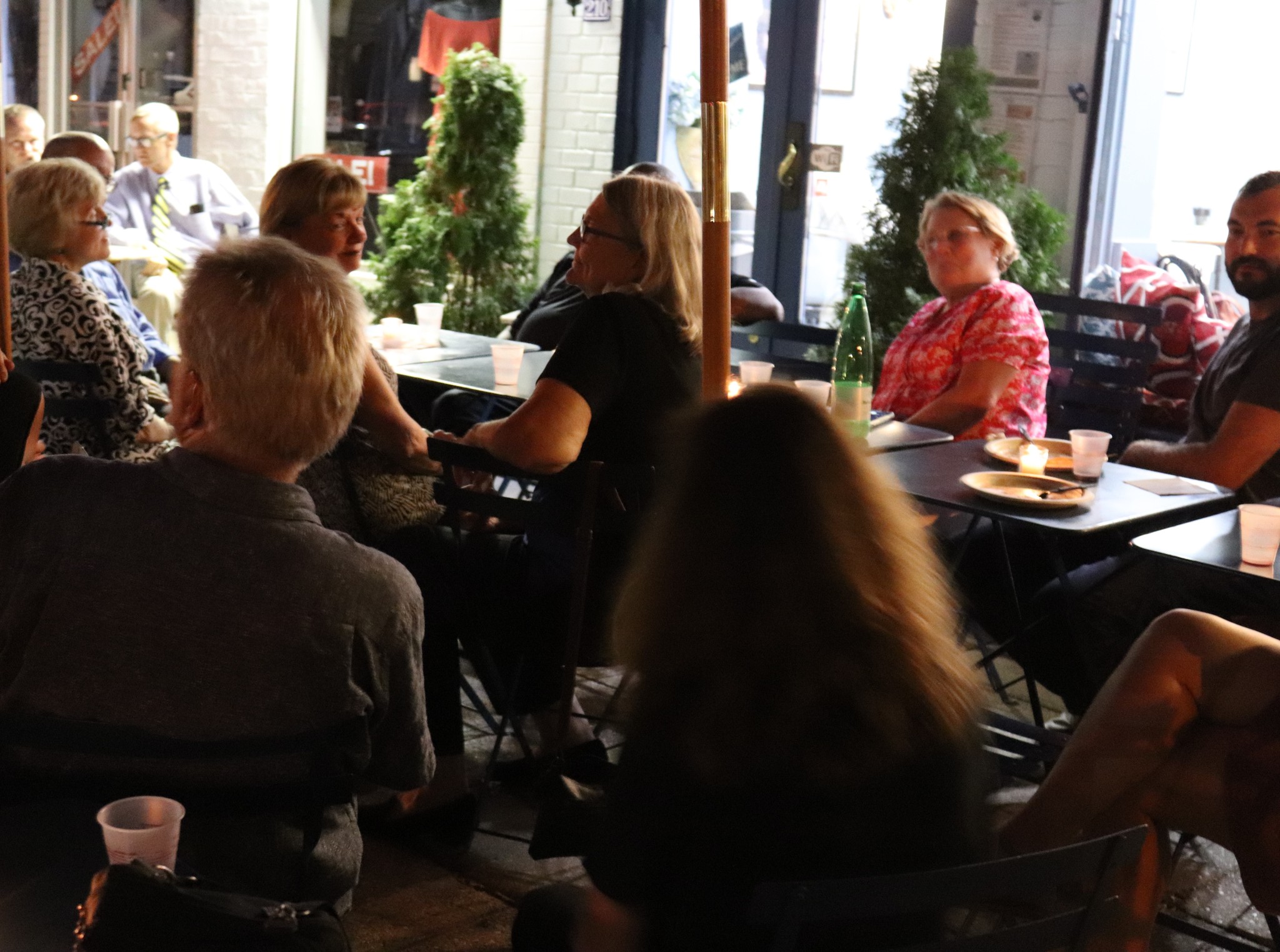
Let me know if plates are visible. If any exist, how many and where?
[959,470,1088,508]
[983,436,1109,471]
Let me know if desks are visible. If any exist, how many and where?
[0,816,512,952]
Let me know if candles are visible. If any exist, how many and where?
[1020,444,1044,475]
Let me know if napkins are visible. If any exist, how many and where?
[1125,477,1211,495]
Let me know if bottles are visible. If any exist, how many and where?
[831,282,873,440]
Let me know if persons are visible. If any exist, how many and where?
[862,190,1050,553]
[0,94,1279,951]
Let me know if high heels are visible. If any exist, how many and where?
[356,785,483,848]
[486,740,609,800]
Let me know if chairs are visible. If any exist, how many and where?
[0,290,1159,952]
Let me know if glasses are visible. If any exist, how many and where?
[580,214,635,248]
[915,225,981,254]
[78,213,114,230]
[125,133,167,148]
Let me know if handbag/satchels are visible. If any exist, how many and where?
[67,858,352,952]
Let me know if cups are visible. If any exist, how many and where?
[380,317,403,348]
[795,379,831,408]
[97,796,187,878]
[740,361,775,386]
[1239,503,1280,566]
[1068,429,1112,483]
[491,343,525,385]
[414,303,446,346]
[1018,443,1049,476]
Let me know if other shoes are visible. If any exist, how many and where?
[952,828,1088,916]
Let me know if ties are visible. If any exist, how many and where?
[151,174,187,276]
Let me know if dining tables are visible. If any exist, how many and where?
[1099,507,1280,875]
[398,341,554,503]
[357,322,537,366]
[862,436,1236,779]
